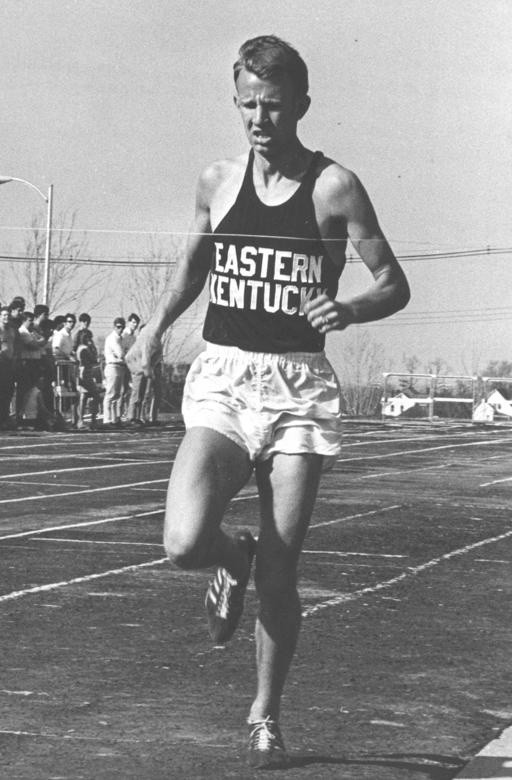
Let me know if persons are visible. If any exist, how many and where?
[0,295,163,430]
[120,34,410,772]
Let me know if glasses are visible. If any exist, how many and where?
[116,325,125,329]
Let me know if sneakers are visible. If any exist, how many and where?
[203,532,254,644]
[73,419,161,430]
[247,719,288,770]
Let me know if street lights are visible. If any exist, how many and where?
[0,173,55,321]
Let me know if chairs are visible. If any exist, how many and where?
[52,359,97,424]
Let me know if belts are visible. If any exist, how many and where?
[109,362,123,366]
